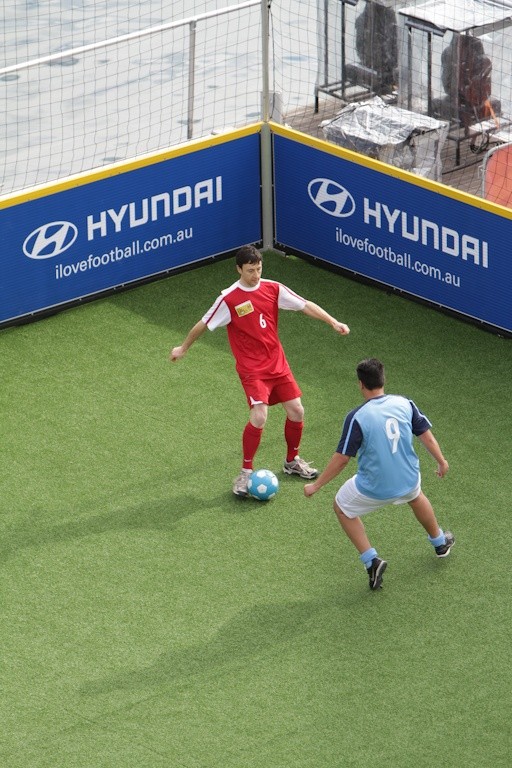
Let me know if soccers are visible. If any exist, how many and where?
[247,469,280,502]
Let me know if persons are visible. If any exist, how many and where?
[304,360,454,591]
[169,244,351,498]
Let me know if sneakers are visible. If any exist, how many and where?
[284,456,318,479]
[366,557,388,590]
[233,471,252,495]
[434,532,456,558]
